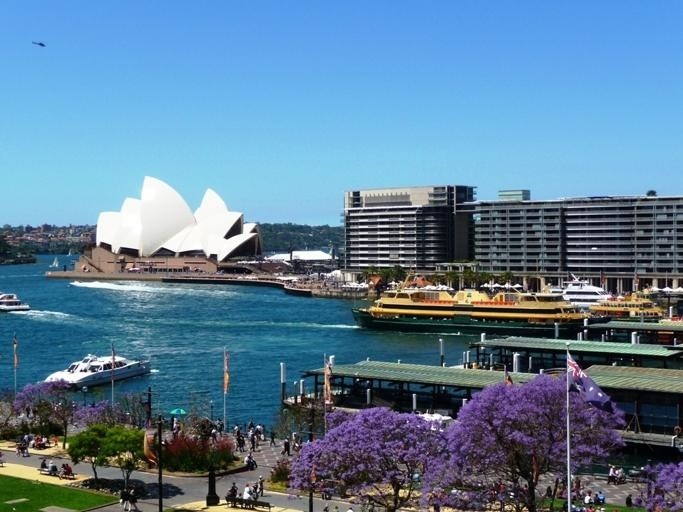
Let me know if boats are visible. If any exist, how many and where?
[1,290,35,315]
[44,350,154,394]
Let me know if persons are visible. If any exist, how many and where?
[15,418,290,512]
[626,494,632,507]
[490,465,624,512]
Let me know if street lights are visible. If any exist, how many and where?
[209,398,217,422]
[81,384,92,408]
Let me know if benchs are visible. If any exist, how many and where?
[225,495,274,512]
[0,460,4,467]
[42,440,51,448]
[37,467,78,480]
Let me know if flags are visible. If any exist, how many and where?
[567,352,617,414]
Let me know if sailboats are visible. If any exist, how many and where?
[47,256,61,270]
[66,249,74,258]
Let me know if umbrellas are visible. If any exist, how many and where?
[170,408,186,415]
